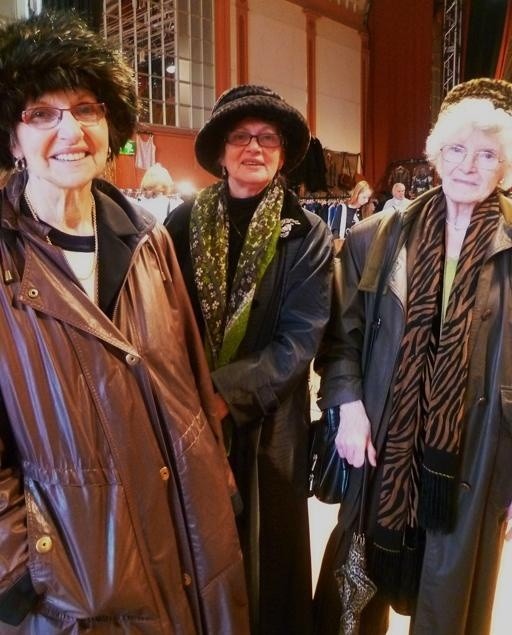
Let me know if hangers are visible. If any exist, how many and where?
[396,165,404,170]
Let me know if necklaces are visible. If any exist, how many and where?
[21,191,100,281]
[444,219,468,232]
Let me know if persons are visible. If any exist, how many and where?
[383,183,413,209]
[359,202,376,219]
[0,2,250,634]
[312,77,512,634]
[162,84,337,633]
[372,185,393,215]
[129,161,186,222]
[328,179,373,242]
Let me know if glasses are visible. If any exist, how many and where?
[436,145,506,169]
[225,131,285,147]
[22,102,107,130]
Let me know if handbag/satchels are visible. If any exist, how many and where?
[310,404,349,503]
[334,239,345,254]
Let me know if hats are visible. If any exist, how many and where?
[437,78,512,120]
[195,85,312,179]
